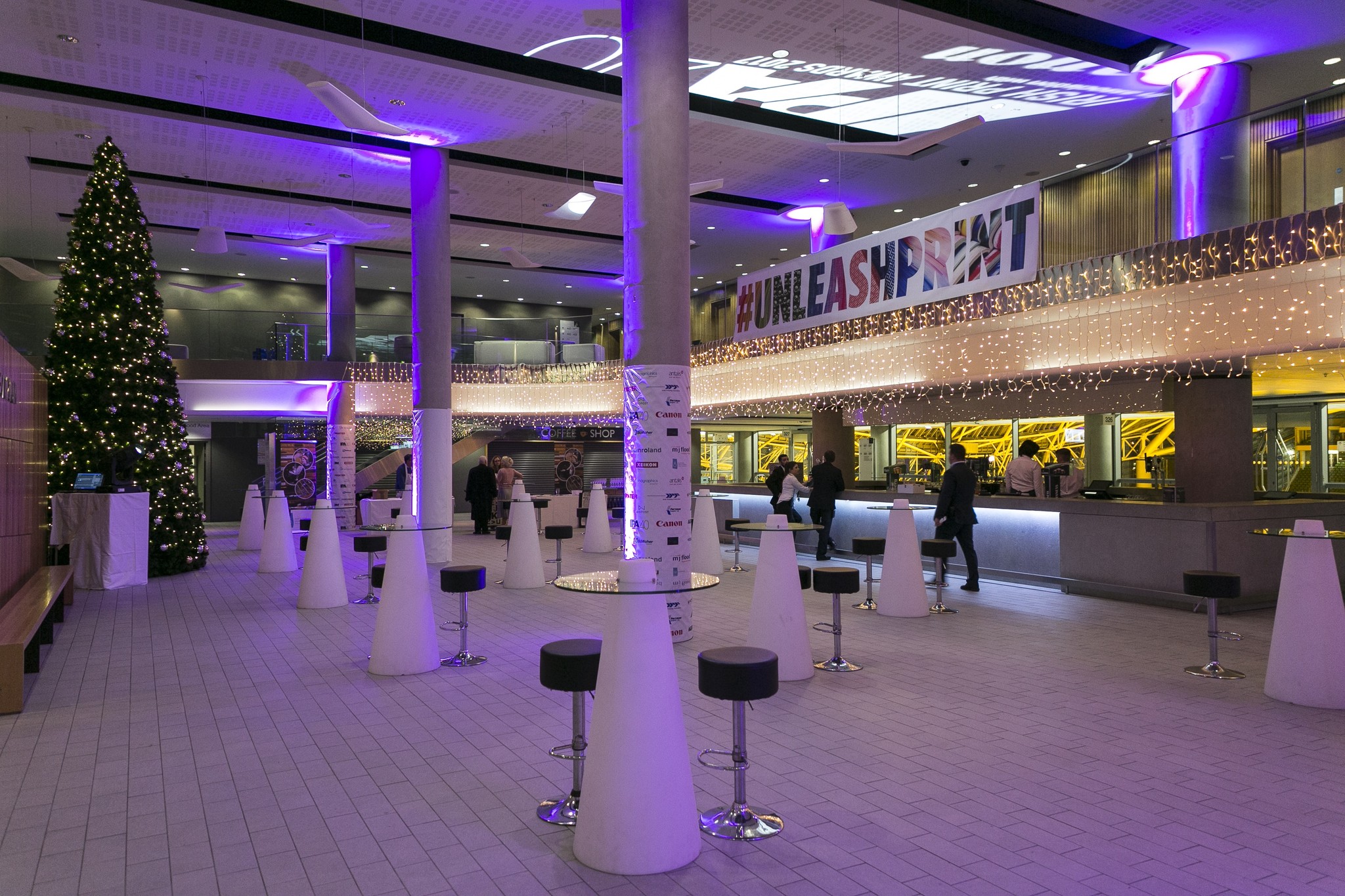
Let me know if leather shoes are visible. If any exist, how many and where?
[961,583,979,592]
[816,555,831,561]
[829,541,836,551]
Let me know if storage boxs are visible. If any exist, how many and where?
[897,485,925,494]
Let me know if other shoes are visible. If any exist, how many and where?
[490,527,495,530]
[473,532,482,534]
[482,531,490,534]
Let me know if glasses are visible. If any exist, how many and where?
[494,460,500,462]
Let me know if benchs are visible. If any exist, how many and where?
[0,565,74,715]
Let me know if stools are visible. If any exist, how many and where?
[299,499,958,841]
[1183,570,1247,679]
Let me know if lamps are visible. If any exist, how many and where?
[823,46,857,235]
[553,99,596,220]
[100,443,146,494]
[193,60,228,253]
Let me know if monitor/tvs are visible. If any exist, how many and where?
[73,473,105,489]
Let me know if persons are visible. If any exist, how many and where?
[562,455,579,476]
[931,443,980,592]
[766,454,789,514]
[777,461,813,541]
[807,451,845,561]
[396,454,413,488]
[465,456,523,534]
[1055,448,1084,499]
[1005,439,1044,498]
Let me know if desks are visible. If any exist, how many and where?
[1245,519,1345,710]
[563,344,605,364]
[164,344,189,359]
[50,493,149,590]
[232,480,936,875]
[474,341,556,365]
